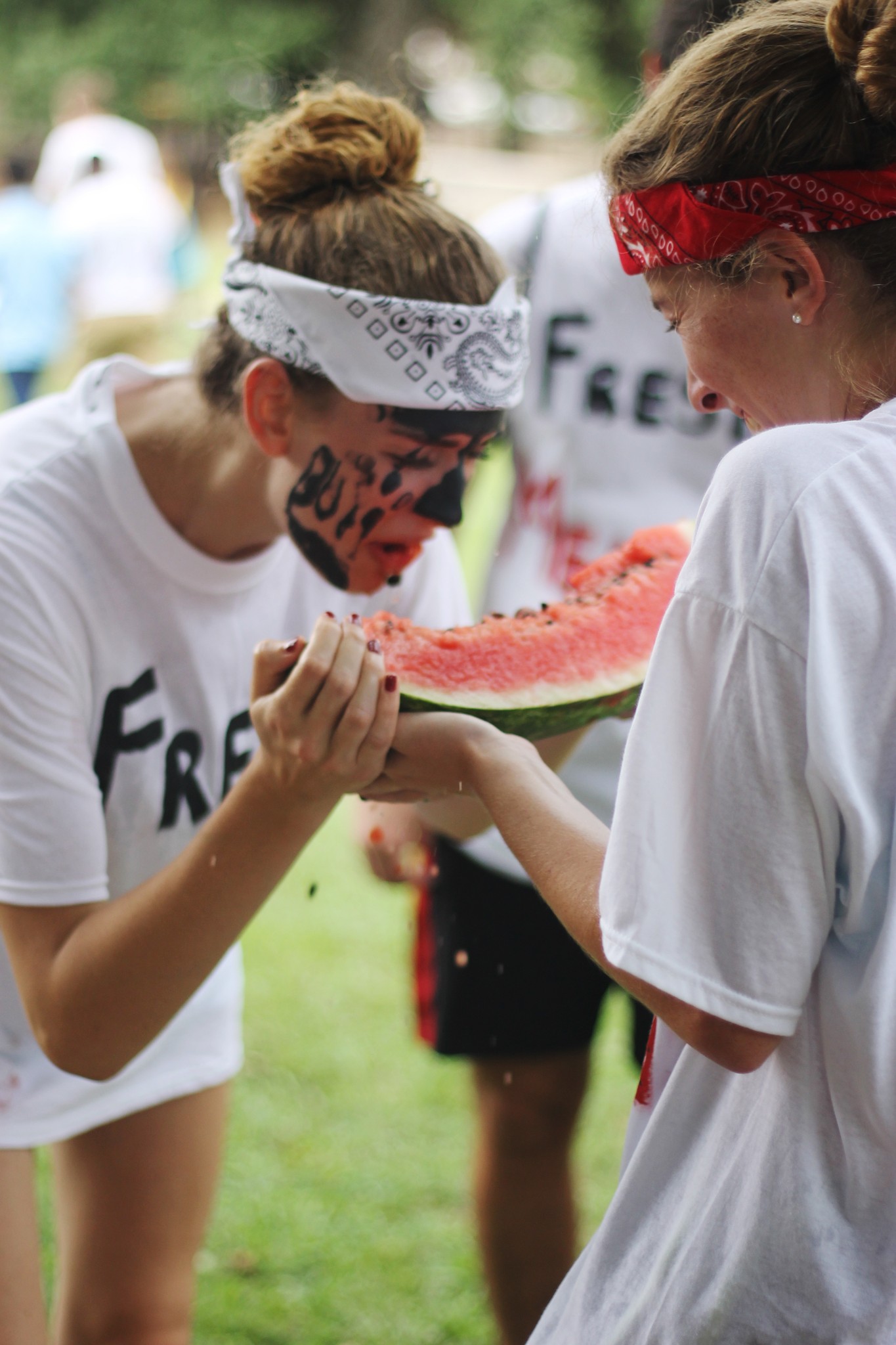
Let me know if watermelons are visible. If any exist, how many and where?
[354,523,694,737]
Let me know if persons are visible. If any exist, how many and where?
[358,1,896,1345]
[365,0,760,1345]
[0,66,184,419]
[0,87,524,1345]
